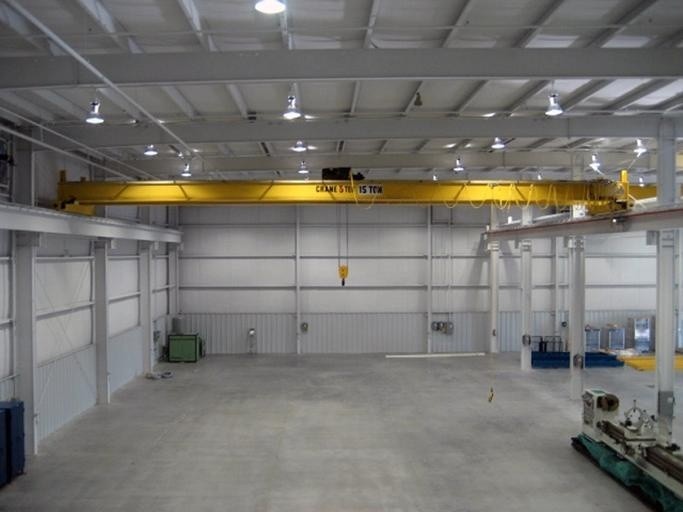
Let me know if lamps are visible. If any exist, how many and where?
[492,136,504,149]
[84,97,106,125]
[453,157,464,172]
[296,161,310,173]
[587,153,601,168]
[634,138,647,153]
[144,144,160,155]
[545,90,564,117]
[283,95,301,119]
[253,0,287,15]
[292,139,307,152]
[182,159,192,178]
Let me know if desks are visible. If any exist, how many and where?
[168,332,199,362]
